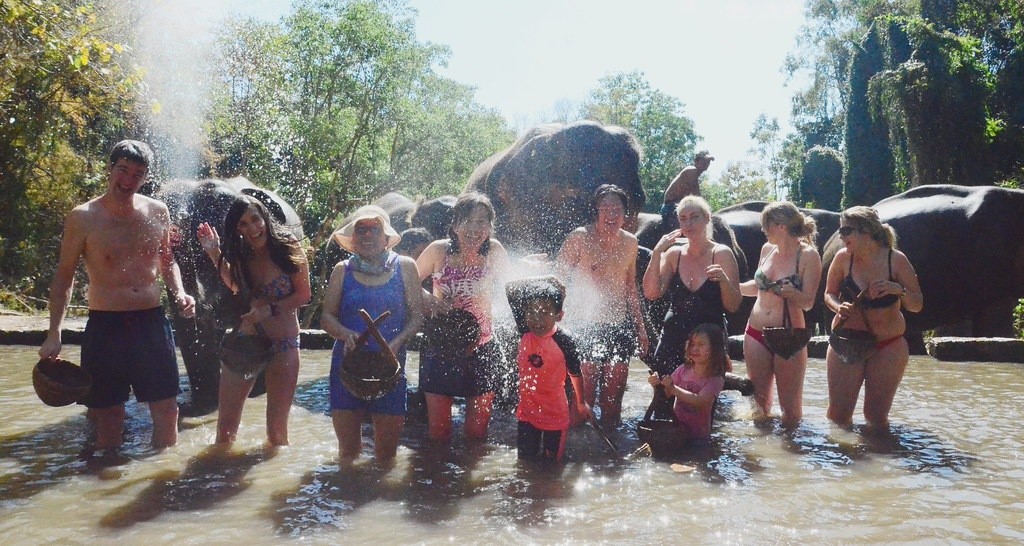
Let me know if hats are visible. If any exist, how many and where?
[694,150,714,161]
[334,205,401,254]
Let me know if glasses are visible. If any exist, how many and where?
[354,226,381,236]
[839,226,857,237]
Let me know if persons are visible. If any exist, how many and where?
[824,205,923,430]
[394,227,436,318]
[196,194,312,445]
[321,205,422,460]
[638,315,754,431]
[642,194,741,436]
[738,201,822,423]
[505,275,592,466]
[662,149,718,242]
[649,320,725,463]
[555,183,649,429]
[38,139,196,449]
[415,190,552,442]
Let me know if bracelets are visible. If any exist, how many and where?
[270,301,281,316]
[900,284,906,299]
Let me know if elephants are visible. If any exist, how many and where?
[155,175,306,395]
[635,211,755,397]
[817,184,1024,350]
[326,192,459,296]
[461,120,645,262]
[715,201,842,328]
